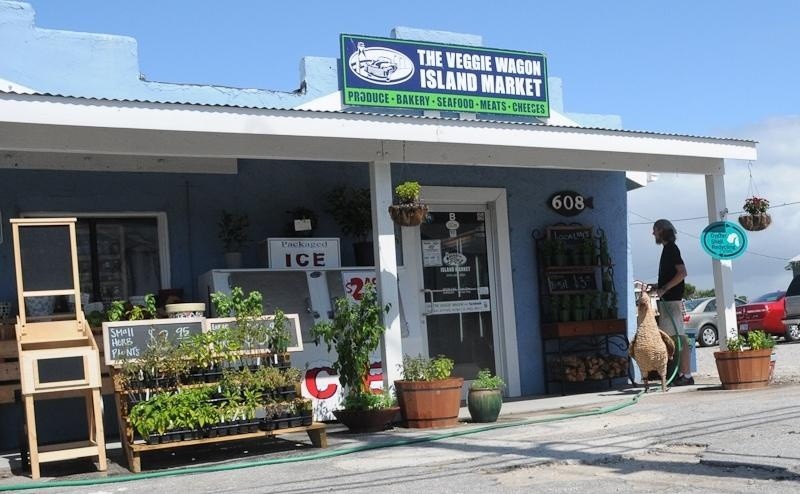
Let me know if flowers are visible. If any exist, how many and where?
[739,197,772,215]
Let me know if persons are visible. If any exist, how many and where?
[647,220,694,386]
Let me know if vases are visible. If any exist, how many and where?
[738,213,772,230]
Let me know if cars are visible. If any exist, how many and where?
[736,291,800,342]
[683,297,748,347]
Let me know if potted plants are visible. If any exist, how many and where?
[538,239,617,322]
[468,368,507,422]
[715,328,776,391]
[308,282,401,433]
[127,287,312,444]
[388,180,428,228]
[217,209,251,268]
[394,352,464,429]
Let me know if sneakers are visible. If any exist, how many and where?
[670,375,694,386]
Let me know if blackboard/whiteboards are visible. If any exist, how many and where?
[102,314,304,366]
[546,227,594,245]
[547,272,602,295]
[10,217,80,297]
[77,224,128,303]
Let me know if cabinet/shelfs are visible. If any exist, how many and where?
[112,355,328,474]
[533,223,636,393]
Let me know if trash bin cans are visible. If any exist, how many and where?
[684,329,700,373]
